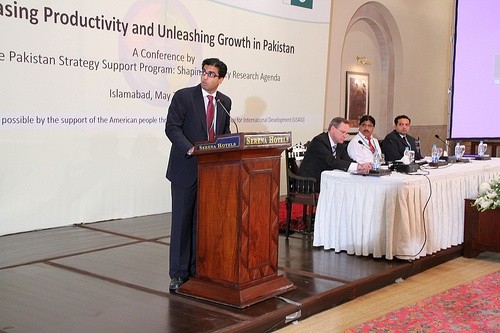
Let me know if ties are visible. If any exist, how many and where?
[206,95,215,140]
[333,145,336,158]
[367,137,375,153]
[403,137,410,150]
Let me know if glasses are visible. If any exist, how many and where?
[200,71,219,78]
[334,125,349,137]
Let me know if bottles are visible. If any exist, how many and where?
[455,142,462,160]
[431,144,438,163]
[372,150,381,171]
[477,140,484,157]
[404,147,411,165]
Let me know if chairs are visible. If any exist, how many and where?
[286,141,319,239]
[471,141,500,157]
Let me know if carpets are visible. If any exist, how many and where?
[342,268,500,333]
[280,200,316,232]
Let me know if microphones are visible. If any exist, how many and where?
[214,97,238,133]
[358,140,391,176]
[435,134,469,163]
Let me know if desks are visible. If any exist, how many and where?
[463,197,500,259]
[312,157,500,260]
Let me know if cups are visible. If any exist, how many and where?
[460,145,465,157]
[410,150,415,162]
[483,144,487,153]
[436,148,443,159]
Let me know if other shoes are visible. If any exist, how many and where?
[169,278,183,289]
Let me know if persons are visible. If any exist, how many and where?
[296,115,421,193]
[165,58,232,290]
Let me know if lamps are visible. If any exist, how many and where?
[357,56,370,65]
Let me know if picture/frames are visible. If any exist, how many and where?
[346,70,371,135]
